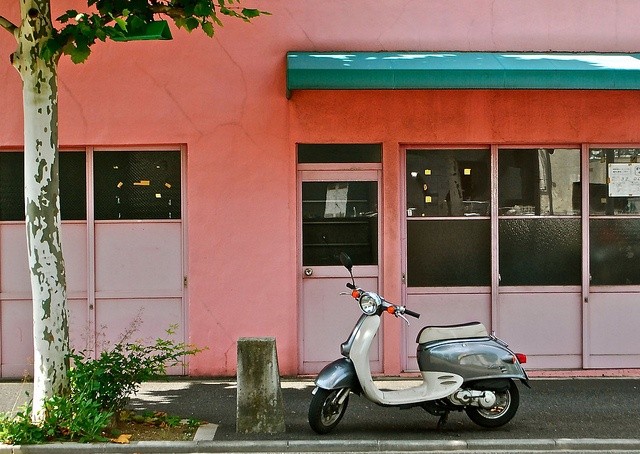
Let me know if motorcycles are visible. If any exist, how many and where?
[307,252,531,434]
